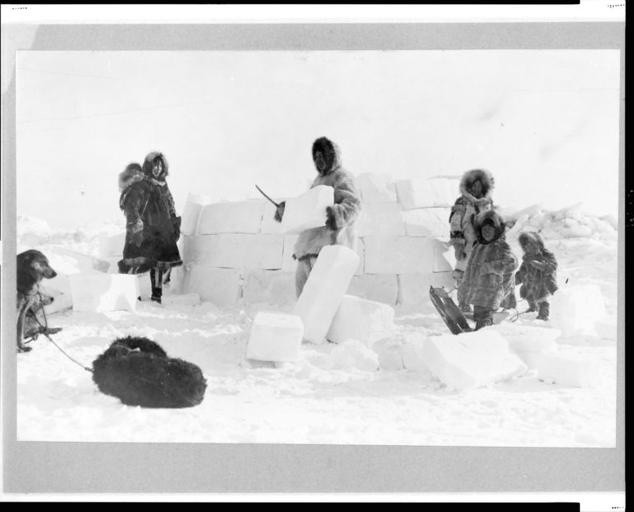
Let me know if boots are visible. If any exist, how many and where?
[526,300,537,313]
[536,302,549,320]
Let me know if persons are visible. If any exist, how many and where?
[118,162,168,248]
[514,228,559,320]
[444,168,520,321]
[116,150,183,303]
[459,209,517,332]
[274,134,364,345]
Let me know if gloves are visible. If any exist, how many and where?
[531,260,545,272]
[453,242,467,261]
[480,262,494,278]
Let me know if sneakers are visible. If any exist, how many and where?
[455,299,519,334]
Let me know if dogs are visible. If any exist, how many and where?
[14,248,63,354]
[92,334,209,409]
